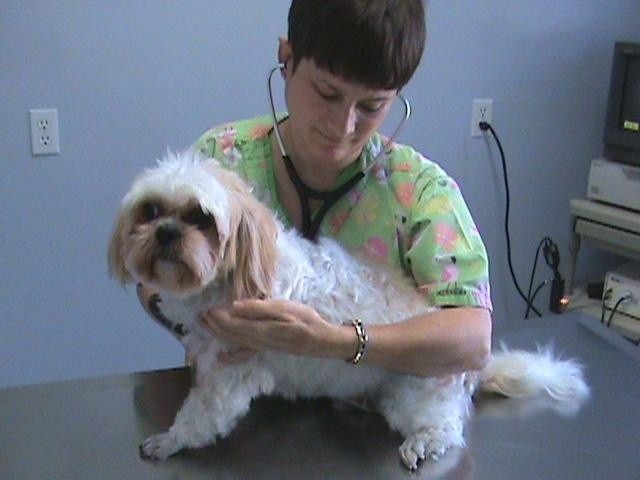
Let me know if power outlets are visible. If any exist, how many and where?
[471,98,493,138]
[30,109,59,155]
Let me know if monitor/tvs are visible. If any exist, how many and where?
[602,41,640,167]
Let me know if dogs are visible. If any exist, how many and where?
[104,145,590,474]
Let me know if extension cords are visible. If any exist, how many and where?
[549,280,563,313]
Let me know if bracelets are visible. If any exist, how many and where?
[340,319,367,366]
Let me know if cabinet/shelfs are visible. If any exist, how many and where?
[563,198,640,344]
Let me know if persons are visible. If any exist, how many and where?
[132,0,495,377]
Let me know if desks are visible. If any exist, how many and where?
[0,297,640,480]
